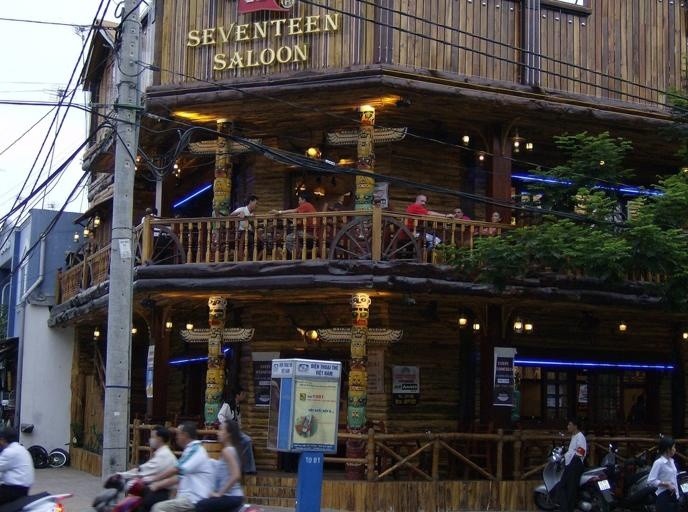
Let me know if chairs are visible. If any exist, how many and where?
[220,204,511,261]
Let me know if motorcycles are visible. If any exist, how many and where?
[106,475,265,512]
[530,430,687,512]
[0,490,73,512]
[89,470,127,512]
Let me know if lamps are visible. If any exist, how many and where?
[456,311,535,335]
[619,319,627,331]
[682,328,688,338]
[459,116,533,161]
[92,315,193,337]
[73,212,102,242]
[305,147,321,158]
[313,185,324,196]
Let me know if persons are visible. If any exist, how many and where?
[1,426,34,506]
[84,229,97,286]
[455,209,470,222]
[272,193,316,260]
[230,195,262,260]
[564,413,587,509]
[491,212,503,224]
[400,194,454,254]
[117,384,257,512]
[647,435,680,512]
[142,208,166,246]
